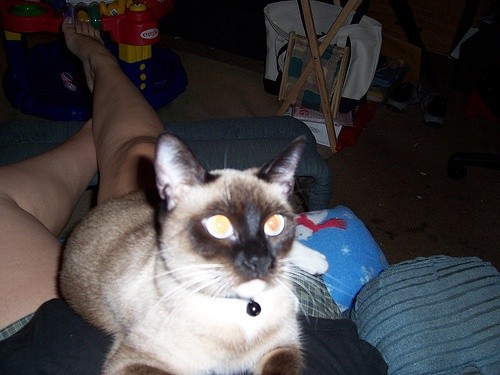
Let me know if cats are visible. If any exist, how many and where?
[51,129,346,375]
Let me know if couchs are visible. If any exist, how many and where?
[0,114,391,375]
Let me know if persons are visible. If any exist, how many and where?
[0,17,389,375]
